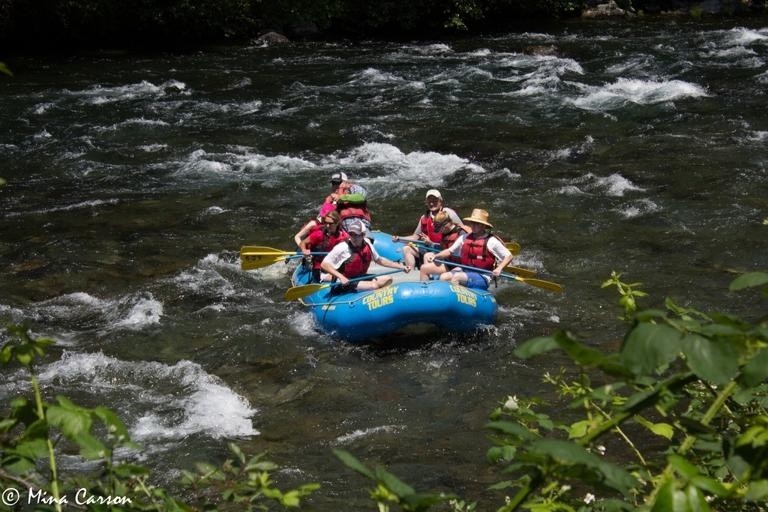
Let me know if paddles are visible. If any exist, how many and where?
[240,257,308,273]
[414,244,538,280]
[432,258,565,294]
[240,246,331,261]
[286,270,404,302]
[396,236,520,255]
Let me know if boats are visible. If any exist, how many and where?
[293,229,499,342]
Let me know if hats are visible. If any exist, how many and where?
[426,189,441,198]
[330,172,347,183]
[463,208,493,229]
[348,220,366,234]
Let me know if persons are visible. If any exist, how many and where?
[419,211,473,281]
[324,172,372,236]
[294,203,335,273]
[392,189,464,271]
[320,220,411,295]
[428,208,513,290]
[299,211,351,283]
